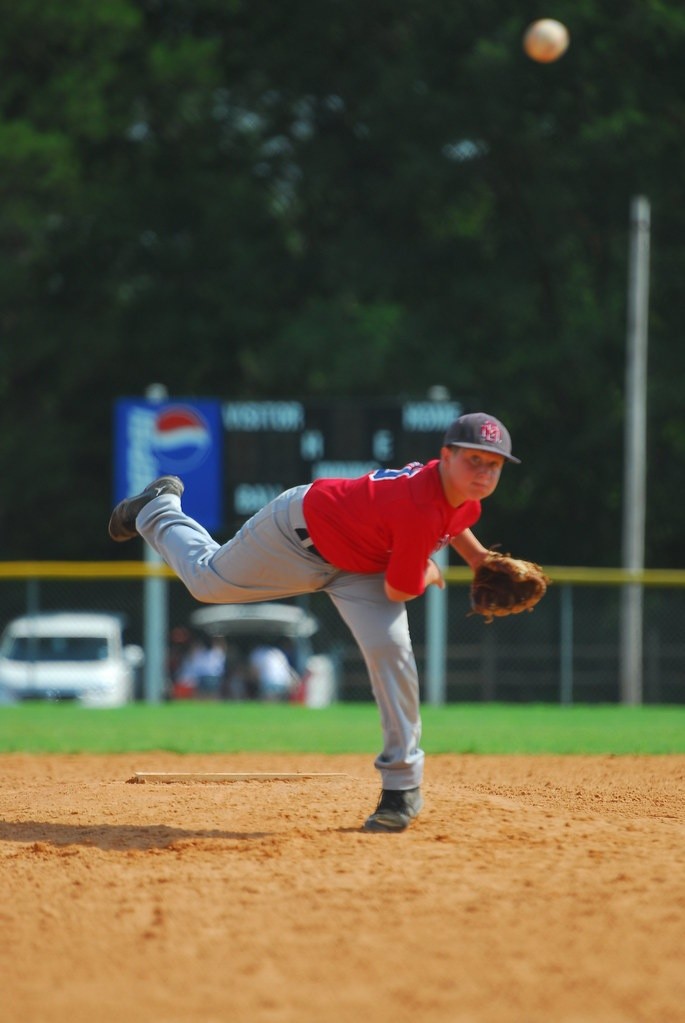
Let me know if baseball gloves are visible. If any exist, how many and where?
[465,543,553,625]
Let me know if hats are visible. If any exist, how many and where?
[443,413,521,465]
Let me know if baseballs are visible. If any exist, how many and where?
[524,18,569,63]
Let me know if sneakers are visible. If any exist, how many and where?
[364,787,423,834]
[109,476,184,542]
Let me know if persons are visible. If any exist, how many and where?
[109,414,546,833]
[168,629,333,706]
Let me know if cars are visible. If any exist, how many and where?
[0,612,145,709]
[166,601,331,710]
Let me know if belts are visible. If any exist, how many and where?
[296,529,328,564]
